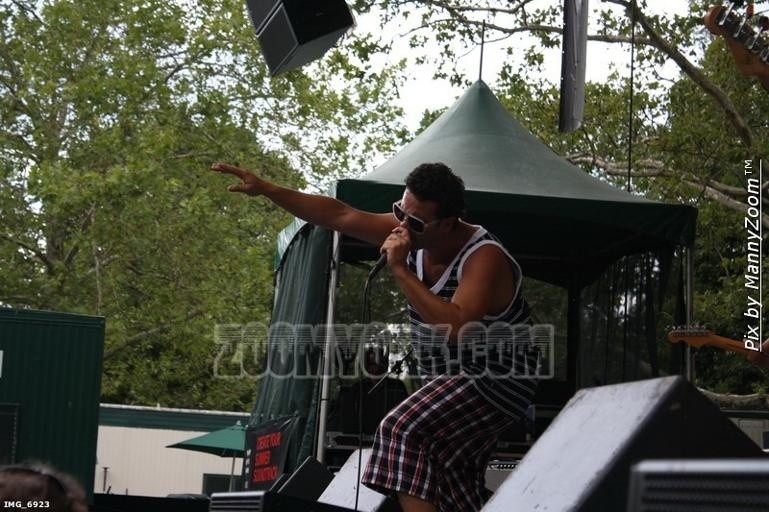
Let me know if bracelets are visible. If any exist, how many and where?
[759,344,765,352]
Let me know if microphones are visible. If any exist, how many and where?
[368,232,401,281]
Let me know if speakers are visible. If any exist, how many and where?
[248,0,354,75]
[242,0,282,35]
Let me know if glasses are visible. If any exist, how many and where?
[392,199,439,236]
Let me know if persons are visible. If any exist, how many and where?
[208,157,546,511]
[0,462,70,511]
[16,461,90,511]
[743,332,769,367]
